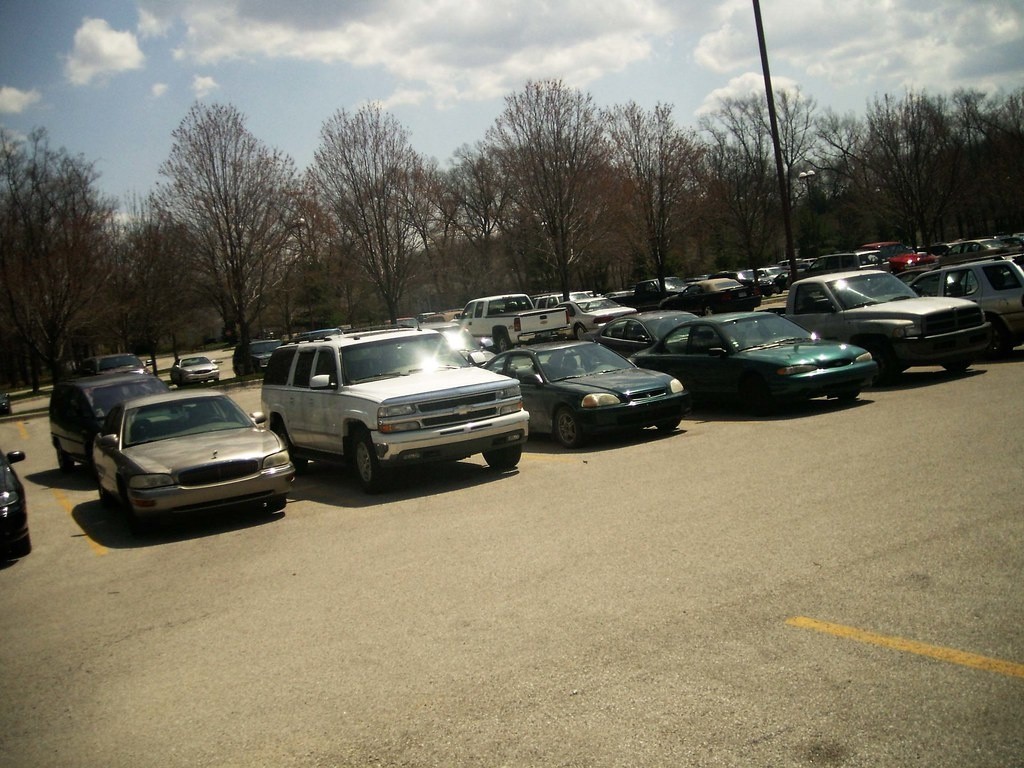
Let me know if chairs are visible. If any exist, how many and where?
[692,331,722,356]
[184,404,225,428]
[129,419,167,442]
[559,356,585,376]
[800,297,824,312]
[504,303,519,313]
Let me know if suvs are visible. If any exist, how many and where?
[49,370,178,476]
[902,252,1024,363]
[260,326,531,495]
[233,339,281,375]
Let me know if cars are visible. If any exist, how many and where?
[0,446,31,556]
[77,351,155,376]
[0,392,10,415]
[627,311,880,415]
[413,321,511,371]
[384,311,448,328]
[676,232,1024,295]
[280,327,344,343]
[659,278,762,320]
[580,310,719,362]
[169,356,220,386]
[528,276,689,341]
[91,388,296,538]
[481,340,692,448]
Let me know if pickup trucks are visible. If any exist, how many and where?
[448,294,572,354]
[754,270,992,384]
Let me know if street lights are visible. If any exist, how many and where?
[798,171,819,253]
